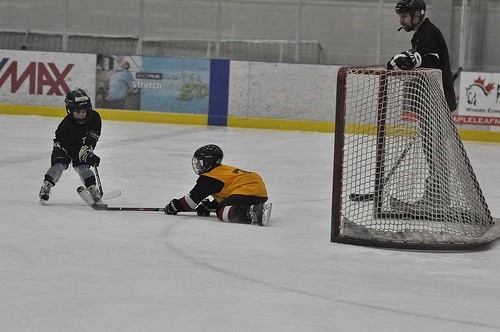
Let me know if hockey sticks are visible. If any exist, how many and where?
[76,185,216,212]
[349,66,463,202]
[89,144,121,200]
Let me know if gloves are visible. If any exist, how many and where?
[80,150,100,167]
[84,132,98,149]
[163,198,180,215]
[197,209,211,216]
[387,50,416,70]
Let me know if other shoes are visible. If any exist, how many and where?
[414,198,452,207]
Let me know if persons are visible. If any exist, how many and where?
[38,88,102,205]
[108,60,138,108]
[388,0,458,206]
[165,144,271,226]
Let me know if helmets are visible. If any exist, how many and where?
[395,0,427,32]
[65,88,92,126]
[192,144,223,175]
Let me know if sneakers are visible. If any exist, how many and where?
[39,180,54,204]
[87,185,104,204]
[251,202,272,225]
